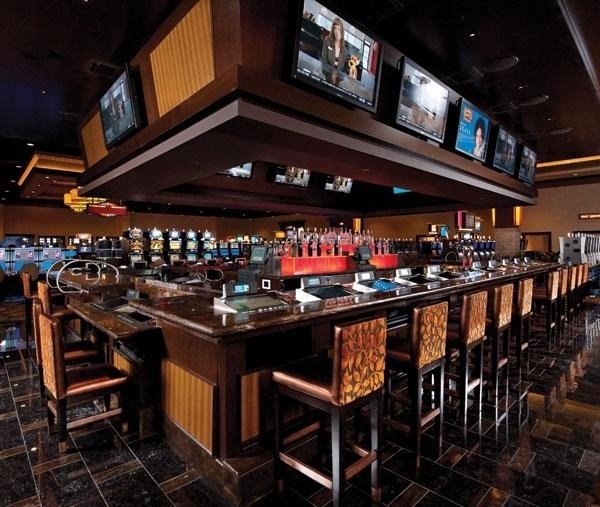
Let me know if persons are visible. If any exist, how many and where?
[108,91,120,128]
[320,18,350,85]
[473,117,486,160]
[413,78,429,126]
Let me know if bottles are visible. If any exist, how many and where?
[268,227,389,256]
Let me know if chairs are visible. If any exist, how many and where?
[39,313,140,453]
[32,299,112,407]
[22,272,57,347]
[37,281,80,320]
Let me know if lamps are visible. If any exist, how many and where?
[64,186,110,213]
[86,196,128,218]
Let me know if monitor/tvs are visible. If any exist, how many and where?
[187,253,197,262]
[204,253,212,261]
[493,123,519,175]
[391,187,413,196]
[86,263,103,269]
[231,249,240,256]
[211,248,218,258]
[130,254,144,266]
[323,174,353,194]
[270,164,312,187]
[517,142,539,186]
[133,261,147,269]
[169,253,180,265]
[216,163,255,180]
[453,98,492,162]
[291,0,385,115]
[98,62,147,150]
[220,248,229,257]
[150,254,162,262]
[357,245,372,261]
[250,246,266,265]
[394,55,449,145]
